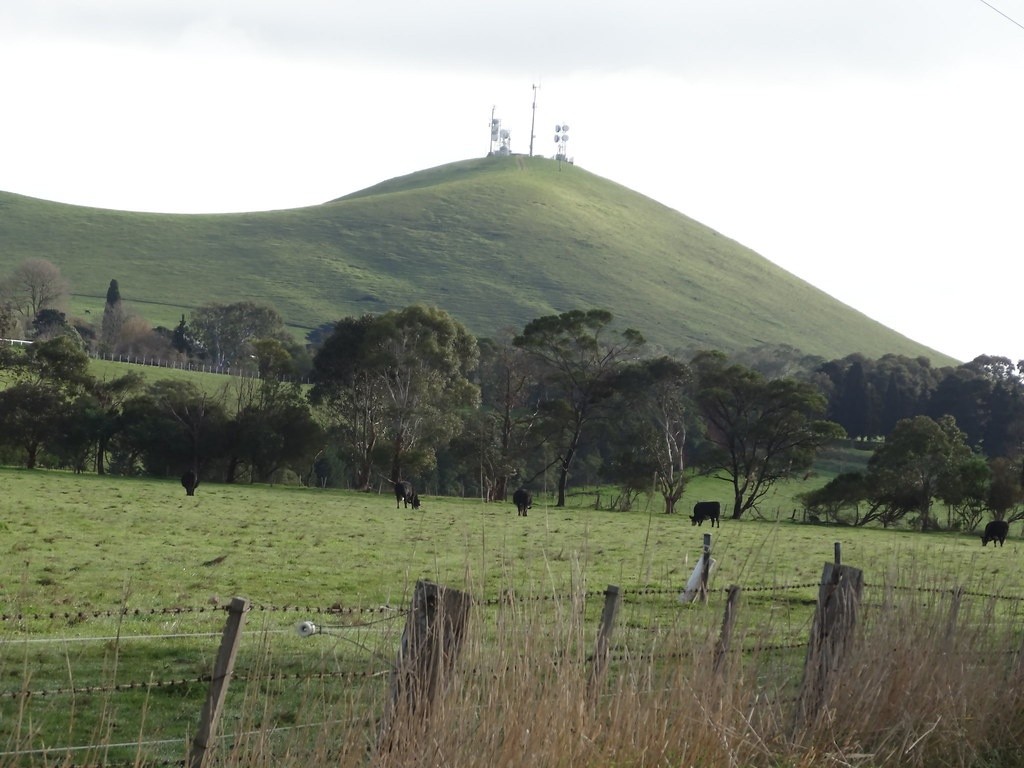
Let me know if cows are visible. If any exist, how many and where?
[181,471,200,496]
[689,501,720,528]
[980,520,1009,547]
[394,480,421,511]
[513,489,532,517]
